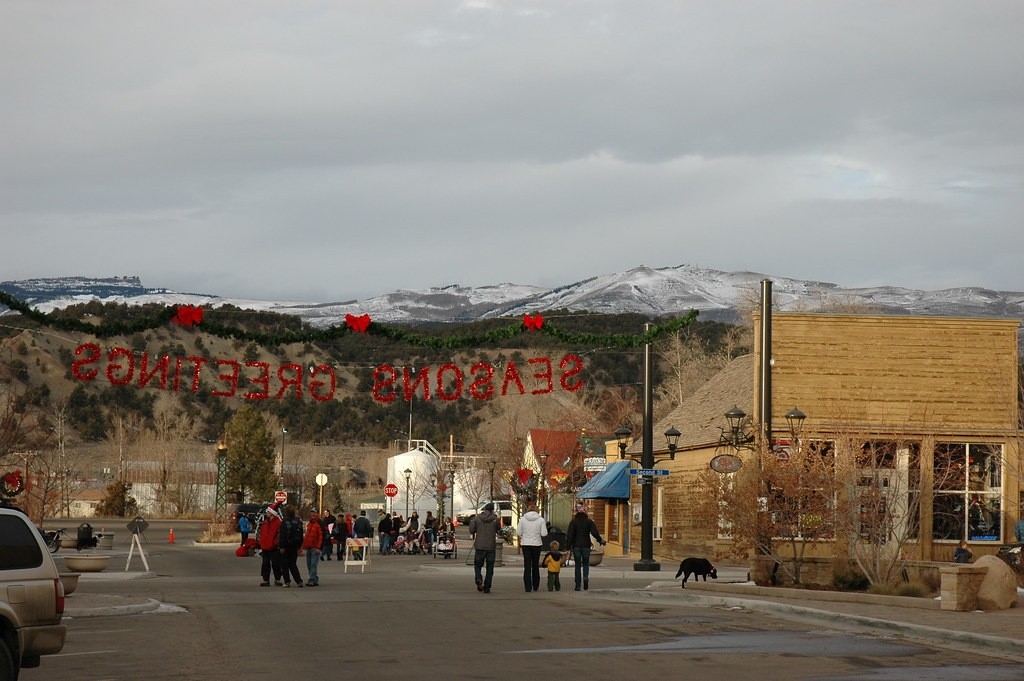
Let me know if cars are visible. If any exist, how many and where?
[0,506,69,681]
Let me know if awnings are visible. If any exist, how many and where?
[577,461,631,498]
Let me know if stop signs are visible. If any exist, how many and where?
[383,483,399,498]
[275,490,288,505]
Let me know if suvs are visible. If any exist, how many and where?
[455,501,513,524]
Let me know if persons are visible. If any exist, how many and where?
[542,542,569,592]
[352,510,373,560]
[321,508,337,561]
[436,517,457,559]
[954,540,972,564]
[514,506,548,591]
[567,512,606,590]
[279,505,304,588]
[257,501,284,587]
[239,511,252,546]
[469,503,502,593]
[303,508,327,587]
[331,515,350,561]
[376,508,437,555]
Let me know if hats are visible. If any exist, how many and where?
[311,507,319,514]
[268,501,282,516]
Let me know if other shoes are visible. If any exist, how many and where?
[297,581,303,587]
[260,580,270,586]
[283,582,290,588]
[306,579,319,586]
[275,579,283,586]
[476,579,483,592]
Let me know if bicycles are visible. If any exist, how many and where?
[42,526,82,554]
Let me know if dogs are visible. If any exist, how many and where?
[674,558,718,588]
[75,533,105,553]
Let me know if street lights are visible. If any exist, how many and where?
[404,468,413,519]
[721,278,809,584]
[448,460,458,530]
[281,428,289,490]
[612,321,682,572]
[430,471,444,524]
[485,458,499,502]
[538,449,551,520]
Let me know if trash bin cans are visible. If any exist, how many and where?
[77,523,93,541]
[425,528,433,554]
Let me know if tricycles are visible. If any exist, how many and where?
[431,531,457,560]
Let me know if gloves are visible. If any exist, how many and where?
[599,539,606,546]
[566,546,570,551]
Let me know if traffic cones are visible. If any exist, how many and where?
[453,514,460,528]
[168,526,175,543]
[500,516,505,530]
